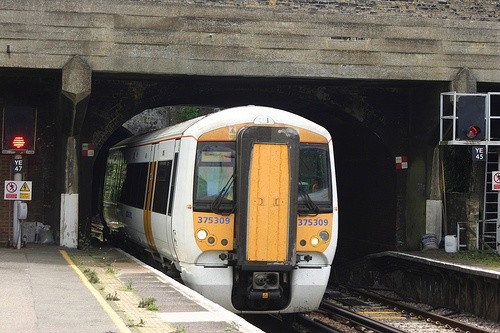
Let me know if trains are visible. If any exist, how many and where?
[100,105,339,315]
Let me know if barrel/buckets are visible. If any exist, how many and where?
[445,235,457,252]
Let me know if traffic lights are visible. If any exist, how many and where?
[3,107,36,155]
[457,96,486,140]
